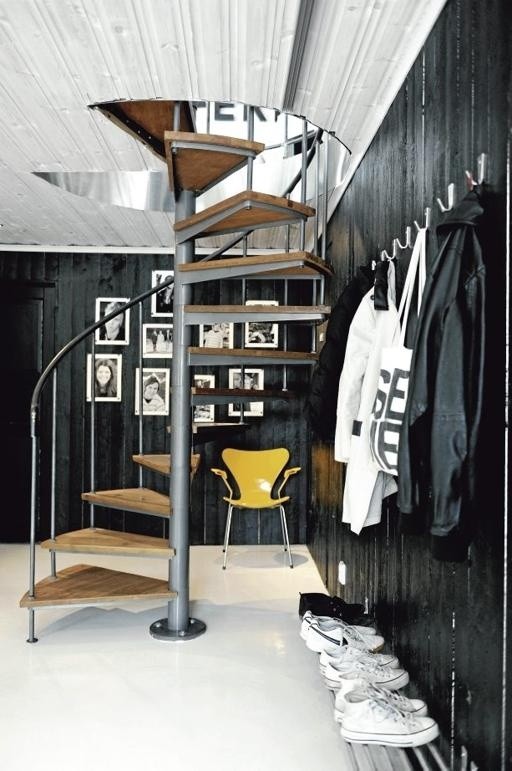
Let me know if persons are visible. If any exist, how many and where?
[204,322,223,349]
[164,329,171,353]
[233,375,251,411]
[156,330,164,353]
[143,374,165,412]
[100,303,124,342]
[95,358,116,398]
[150,329,157,352]
[159,275,174,312]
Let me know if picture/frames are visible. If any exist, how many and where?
[134,366,171,417]
[190,374,217,424]
[244,299,279,350]
[94,295,131,348]
[150,268,176,319]
[198,321,235,350]
[227,368,264,418]
[83,351,122,404]
[140,321,175,360]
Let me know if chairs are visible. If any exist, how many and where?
[209,445,302,572]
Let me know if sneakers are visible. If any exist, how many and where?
[340,688,439,744]
[303,630,387,652]
[332,681,428,720]
[300,611,379,636]
[320,650,400,673]
[329,666,409,690]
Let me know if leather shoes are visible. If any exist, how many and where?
[333,608,376,625]
[299,588,363,613]
